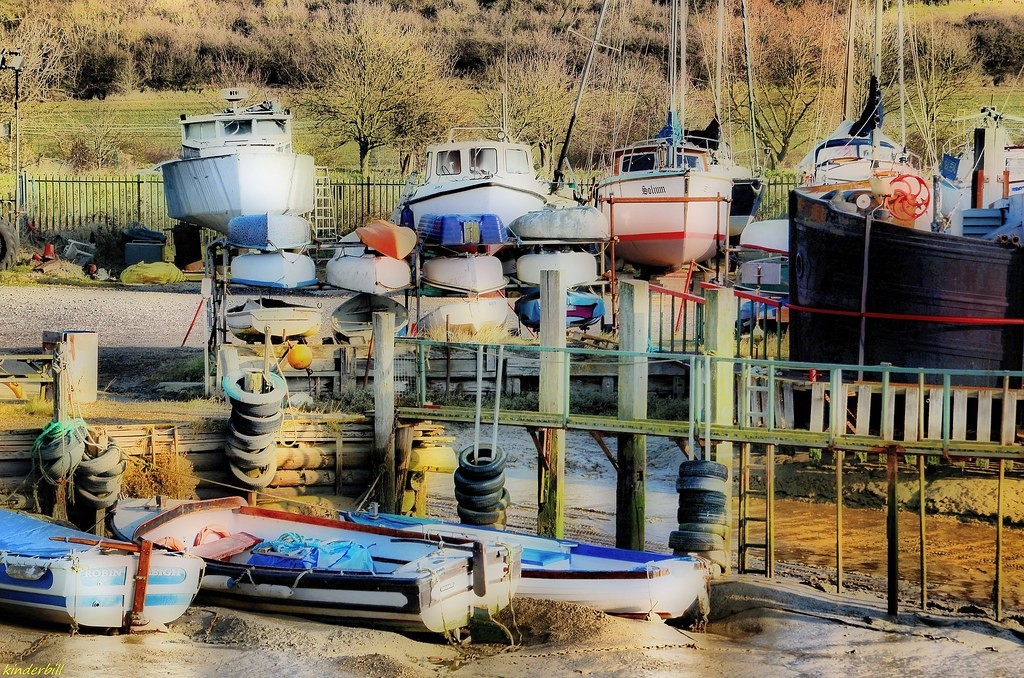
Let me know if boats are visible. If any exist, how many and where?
[151,0,1024,344]
[111,494,524,646]
[788,93,1023,440]
[335,501,711,624]
[0,508,207,637]
